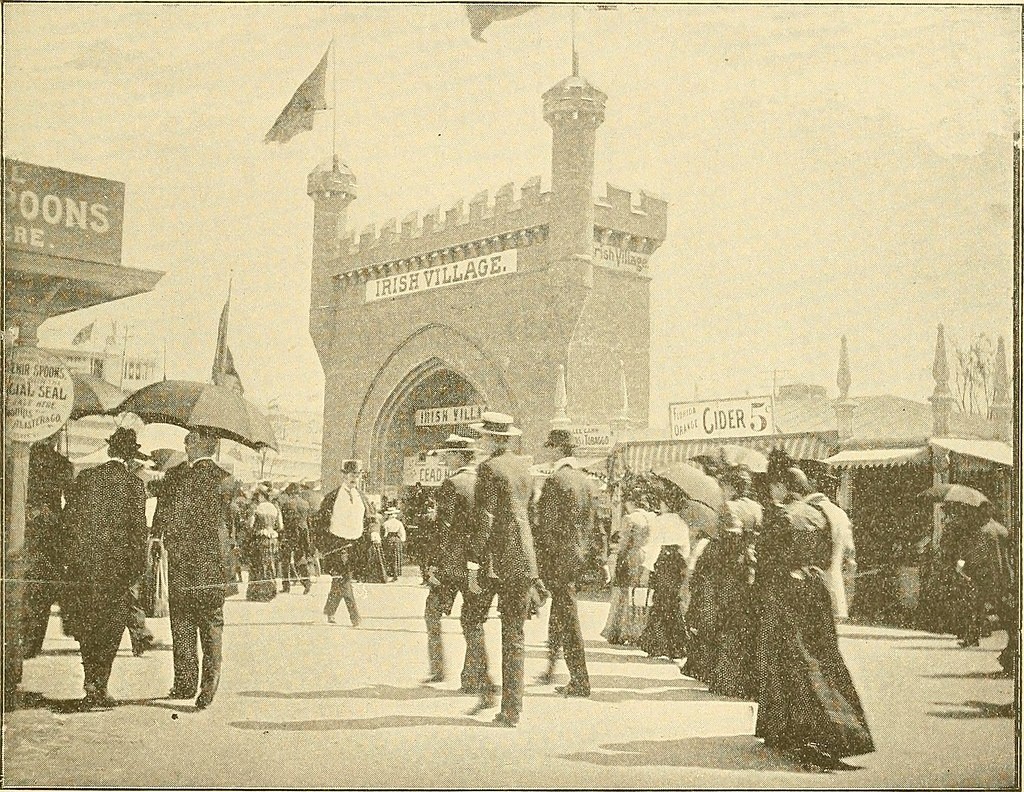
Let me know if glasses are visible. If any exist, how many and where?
[544,442,556,448]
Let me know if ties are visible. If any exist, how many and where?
[346,488,355,506]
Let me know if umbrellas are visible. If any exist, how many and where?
[915,482,992,507]
[115,380,278,453]
[67,372,129,420]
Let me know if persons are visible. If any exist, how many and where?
[1,412,1022,772]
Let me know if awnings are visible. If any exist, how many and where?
[823,446,929,470]
[927,438,1013,467]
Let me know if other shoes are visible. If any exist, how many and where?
[419,673,447,684]
[78,697,95,713]
[556,685,591,697]
[491,715,517,728]
[327,614,361,627]
[132,637,164,656]
[467,700,496,714]
[169,689,196,700]
[279,588,290,592]
[457,681,491,696]
[99,697,124,706]
[533,672,556,684]
[195,696,209,709]
[303,582,312,595]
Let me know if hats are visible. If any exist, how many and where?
[104,427,141,454]
[339,460,365,473]
[384,506,400,514]
[434,436,480,453]
[798,458,837,479]
[470,412,523,436]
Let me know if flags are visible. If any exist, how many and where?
[467,5,536,42]
[72,322,94,345]
[212,300,244,395]
[262,47,327,145]
